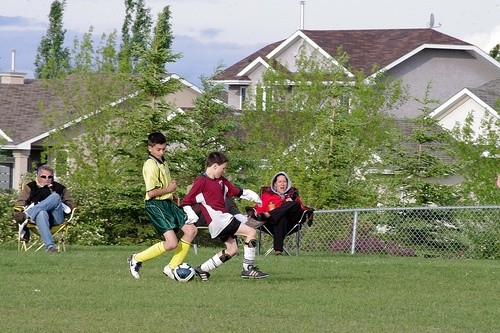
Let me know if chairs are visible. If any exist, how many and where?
[13,182,79,253]
[245,186,315,256]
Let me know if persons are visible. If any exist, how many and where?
[13,165,75,253]
[245,171,306,255]
[127,132,198,279]
[182,152,268,282]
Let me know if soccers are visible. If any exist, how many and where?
[173,263,196,283]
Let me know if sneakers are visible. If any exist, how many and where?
[194,264,210,283]
[241,265,269,279]
[163,264,177,281]
[129,253,143,279]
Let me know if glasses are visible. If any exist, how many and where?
[40,176,53,178]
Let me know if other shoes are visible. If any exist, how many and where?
[253,213,267,222]
[14,212,26,224]
[47,247,59,254]
[274,251,283,256]
